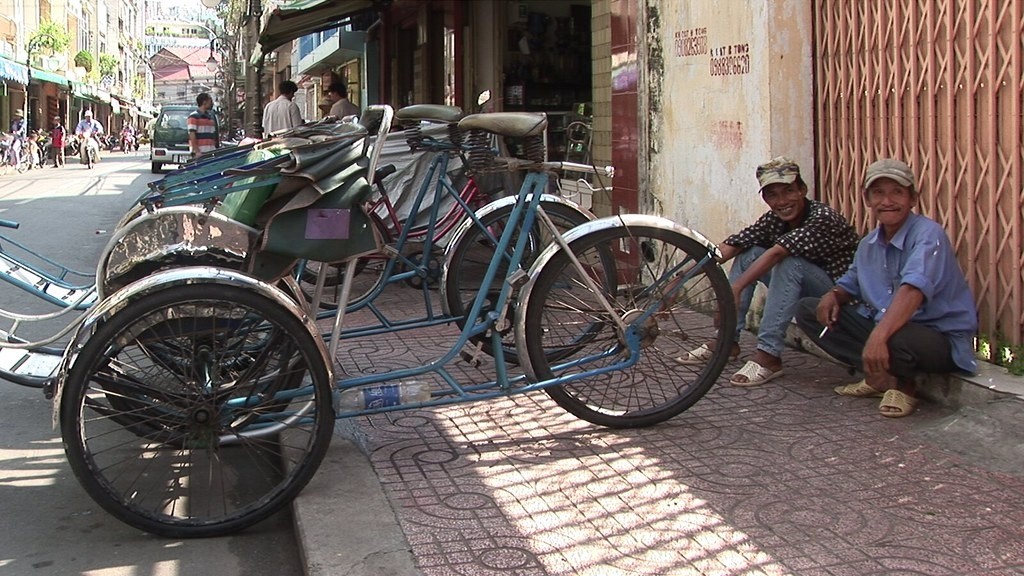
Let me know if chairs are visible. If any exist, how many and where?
[167,119,180,128]
[217,146,279,230]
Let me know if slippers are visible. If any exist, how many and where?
[834,377,885,398]
[729,360,785,386]
[675,343,736,364]
[878,389,920,417]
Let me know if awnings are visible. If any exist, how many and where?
[0,56,121,114]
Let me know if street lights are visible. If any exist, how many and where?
[25,32,60,136]
[206,36,237,139]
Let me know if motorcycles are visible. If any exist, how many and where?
[78,131,102,169]
[118,131,131,155]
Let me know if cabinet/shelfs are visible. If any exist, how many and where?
[545,111,576,157]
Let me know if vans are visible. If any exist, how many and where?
[150,105,220,176]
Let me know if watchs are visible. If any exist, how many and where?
[676,270,688,282]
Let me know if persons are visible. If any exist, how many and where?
[319,96,335,119]
[8,109,25,169]
[648,155,875,387]
[41,116,66,168]
[29,133,39,171]
[328,83,361,119]
[187,93,219,159]
[118,122,136,150]
[76,110,104,161]
[148,113,160,160]
[794,158,978,416]
[262,81,303,133]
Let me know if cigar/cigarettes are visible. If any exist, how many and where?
[819,326,828,339]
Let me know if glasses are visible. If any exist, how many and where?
[329,92,332,95]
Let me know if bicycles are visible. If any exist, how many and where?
[0,128,82,174]
[0,89,738,538]
[97,134,118,151]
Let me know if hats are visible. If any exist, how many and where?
[865,159,913,190]
[755,156,800,194]
[85,110,92,117]
[15,109,24,117]
